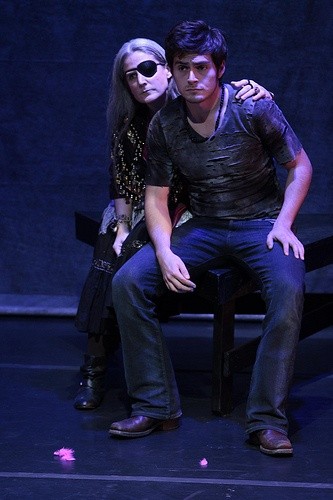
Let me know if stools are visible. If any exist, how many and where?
[75,213,333,417]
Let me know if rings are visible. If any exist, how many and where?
[246,79,260,92]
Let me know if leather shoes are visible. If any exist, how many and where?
[74,364,108,409]
[259,429,294,455]
[109,415,184,437]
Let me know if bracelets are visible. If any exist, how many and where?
[270,91,274,99]
[117,216,130,223]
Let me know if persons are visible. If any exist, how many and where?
[108,18,312,457]
[73,37,277,411]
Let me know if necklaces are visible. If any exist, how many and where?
[185,83,224,143]
